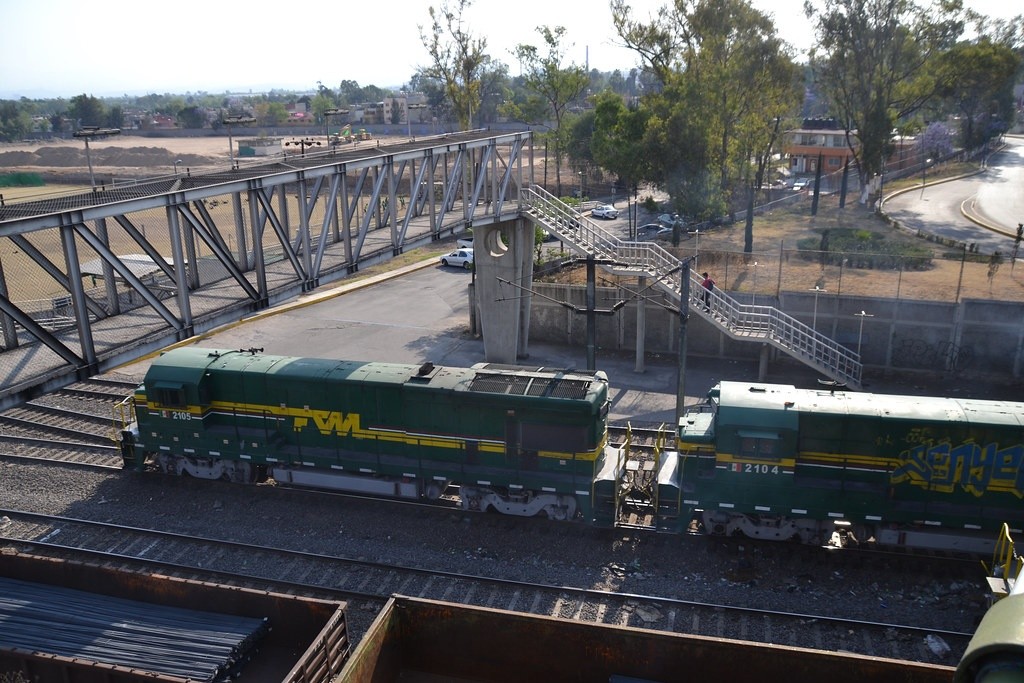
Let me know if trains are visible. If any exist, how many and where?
[112,345,1024,561]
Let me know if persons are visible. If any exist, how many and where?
[697,273,716,314]
[364,194,407,217]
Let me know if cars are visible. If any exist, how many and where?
[439,248,473,270]
[457,238,474,249]
[658,214,687,229]
[591,205,619,220]
[637,224,674,236]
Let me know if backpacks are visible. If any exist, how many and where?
[708,279,713,290]
